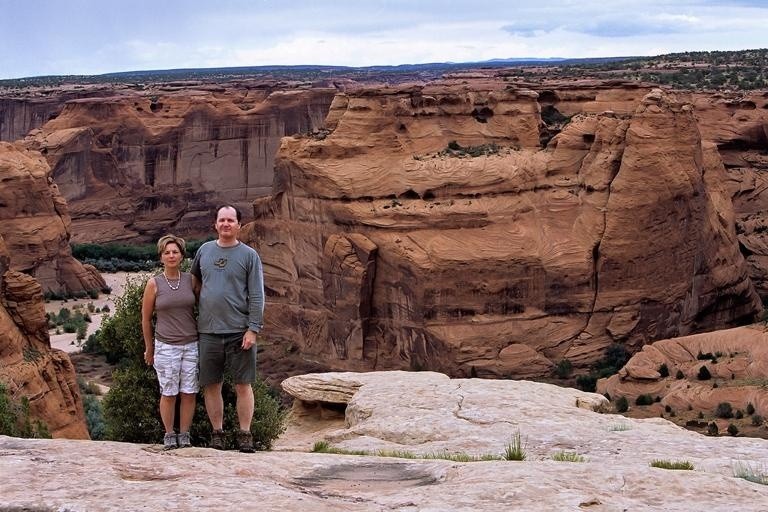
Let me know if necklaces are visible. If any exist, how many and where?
[162,269,182,290]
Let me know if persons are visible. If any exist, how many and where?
[139,232,198,446]
[189,205,264,454]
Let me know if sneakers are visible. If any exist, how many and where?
[164,432,177,450]
[211,429,226,450]
[236,430,256,452]
[179,431,192,448]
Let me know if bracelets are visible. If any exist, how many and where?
[143,352,154,355]
